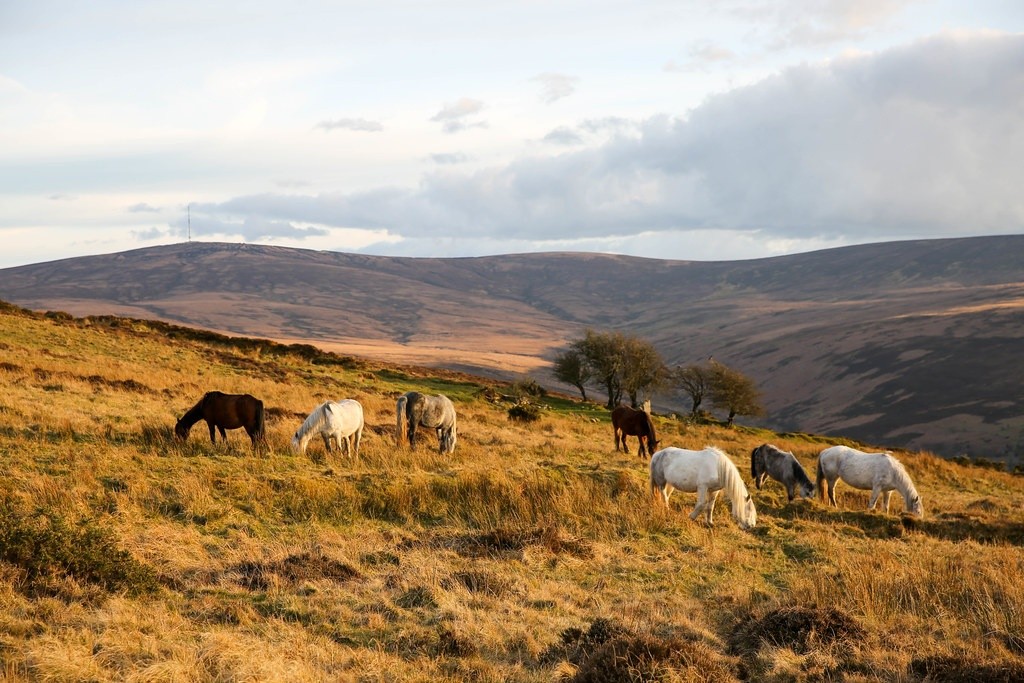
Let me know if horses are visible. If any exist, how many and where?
[815,445,924,519]
[750,443,816,503]
[174,391,265,453]
[293,398,364,459]
[649,446,756,529]
[611,405,661,460]
[395,391,457,455]
